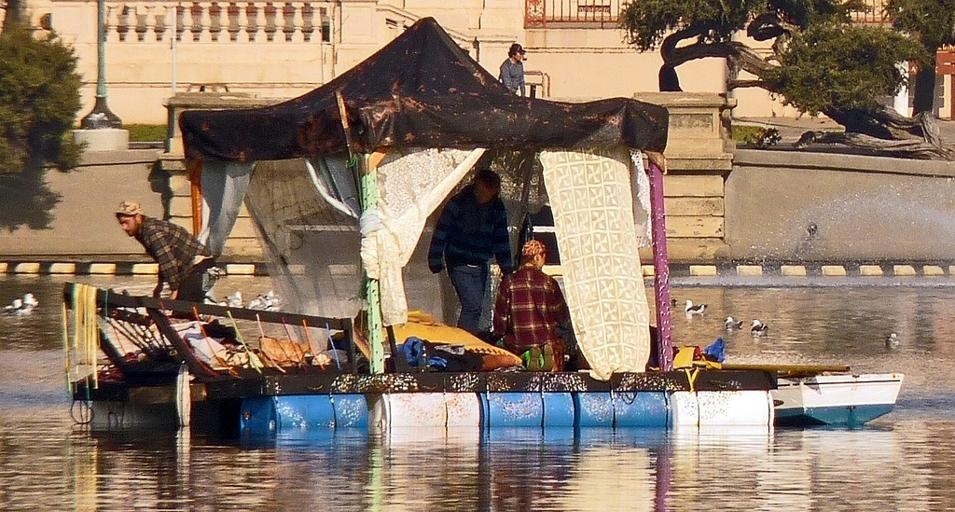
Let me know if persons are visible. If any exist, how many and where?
[112,201,215,320]
[427,168,514,334]
[497,43,526,100]
[493,239,573,372]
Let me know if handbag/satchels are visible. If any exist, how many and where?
[259,335,305,368]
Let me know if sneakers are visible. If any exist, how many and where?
[524,348,541,372]
[539,344,555,372]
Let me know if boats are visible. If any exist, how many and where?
[763,368,906,430]
[68,361,776,442]
[779,431,909,456]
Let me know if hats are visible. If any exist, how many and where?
[113,201,146,217]
[520,240,547,266]
[511,43,526,53]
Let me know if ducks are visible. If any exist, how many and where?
[682,299,709,314]
[671,294,679,306]
[205,287,283,313]
[3,293,35,310]
[750,319,769,337]
[886,333,900,351]
[723,316,744,332]
[8,300,39,315]
